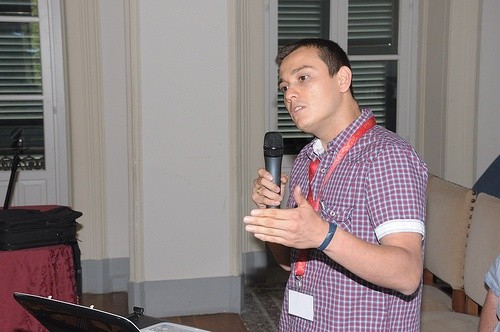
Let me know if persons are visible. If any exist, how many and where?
[241,38,430,332]
[475,254,500,332]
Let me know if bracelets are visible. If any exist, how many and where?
[315,218,337,251]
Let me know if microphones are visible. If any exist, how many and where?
[263,131,284,208]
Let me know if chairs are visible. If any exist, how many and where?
[421,174,500,332]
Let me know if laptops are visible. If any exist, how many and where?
[13,292,213,332]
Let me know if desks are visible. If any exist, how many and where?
[0,206,83,332]
